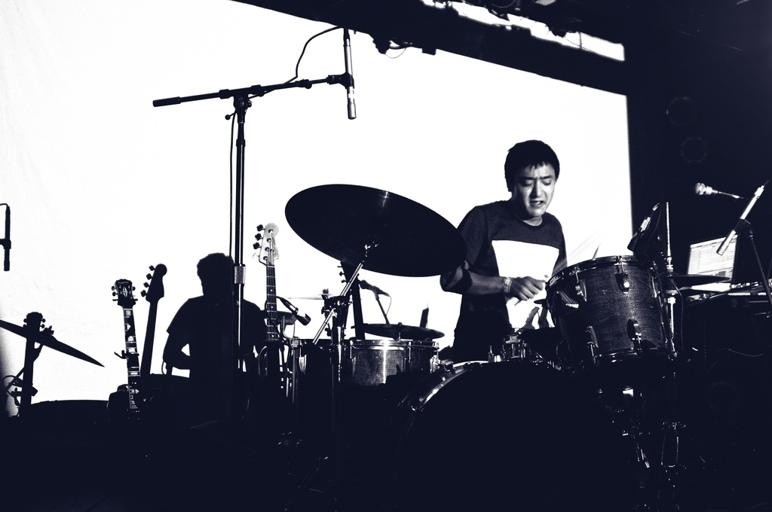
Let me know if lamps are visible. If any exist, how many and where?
[485,0,584,38]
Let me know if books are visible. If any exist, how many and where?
[685,234,739,302]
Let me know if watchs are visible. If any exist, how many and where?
[501,275,516,295]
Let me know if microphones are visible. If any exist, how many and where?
[715,186,765,254]
[693,181,742,204]
[341,30,360,121]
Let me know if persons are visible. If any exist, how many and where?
[162,251,270,380]
[439,139,570,360]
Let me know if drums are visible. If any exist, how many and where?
[384,358,629,512]
[545,256,675,382]
[290,337,436,445]
[502,335,534,359]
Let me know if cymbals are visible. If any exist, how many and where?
[360,324,442,336]
[0,320,105,367]
[659,274,728,290]
[284,185,467,276]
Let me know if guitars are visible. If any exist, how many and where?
[106,265,166,467]
[242,223,296,448]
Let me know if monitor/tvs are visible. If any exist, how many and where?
[685,237,738,285]
